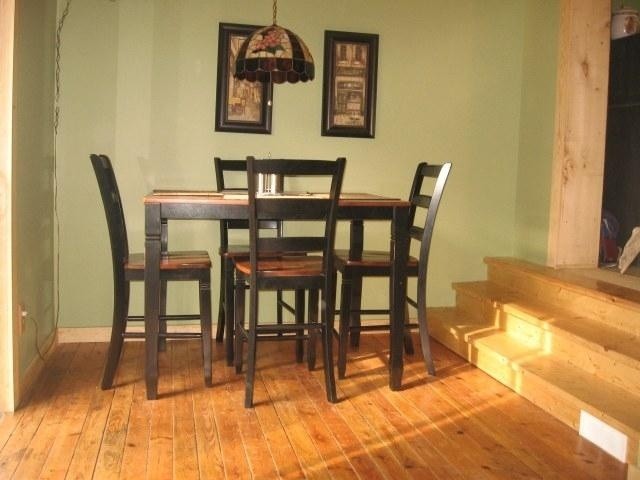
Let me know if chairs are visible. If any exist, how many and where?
[213,157,305,340]
[321,162,451,377]
[88,153,211,392]
[233,153,347,408]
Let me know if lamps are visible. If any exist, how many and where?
[234,0,315,84]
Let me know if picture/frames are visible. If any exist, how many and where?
[320,30,379,138]
[214,20,275,135]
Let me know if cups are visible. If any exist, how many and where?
[257,172,278,200]
